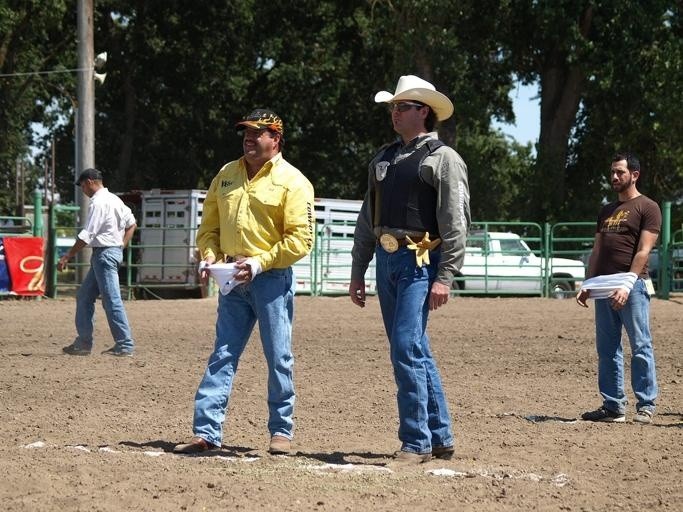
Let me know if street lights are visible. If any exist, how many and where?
[12,73,94,290]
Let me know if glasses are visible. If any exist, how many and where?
[388,101,424,113]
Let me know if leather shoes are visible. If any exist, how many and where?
[385,450,433,467]
[268,434,296,455]
[171,435,222,455]
[432,444,455,459]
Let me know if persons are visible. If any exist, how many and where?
[574,153,662,426]
[55,168,137,356]
[349,74,473,464]
[172,109,315,457]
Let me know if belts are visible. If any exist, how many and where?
[223,254,246,263]
[374,233,438,254]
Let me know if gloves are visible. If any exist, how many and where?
[404,232,442,270]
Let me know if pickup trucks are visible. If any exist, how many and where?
[445,231,587,301]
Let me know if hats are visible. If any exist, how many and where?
[234,108,285,137]
[373,73,455,122]
[72,168,104,187]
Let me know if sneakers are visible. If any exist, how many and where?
[580,404,628,424]
[102,343,134,357]
[61,341,95,358]
[632,408,653,425]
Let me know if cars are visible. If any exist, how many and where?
[580,242,682,283]
[577,243,682,291]
